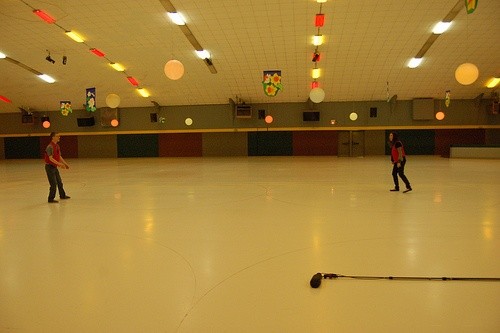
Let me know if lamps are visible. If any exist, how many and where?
[32,10,150,128]
[164,12,210,80]
[407,21,479,85]
[349,112,358,120]
[436,112,444,120]
[330,120,335,124]
[185,118,193,125]
[309,0,327,103]
[486,77,500,89]
[265,116,273,124]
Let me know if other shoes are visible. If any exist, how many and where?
[60,196,71,199]
[48,199,58,202]
[403,188,412,193]
[390,189,399,191]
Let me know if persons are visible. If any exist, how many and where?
[44,131,71,203]
[389,131,412,193]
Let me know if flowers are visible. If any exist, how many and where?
[262,73,282,96]
[59,103,72,117]
[85,92,97,112]
